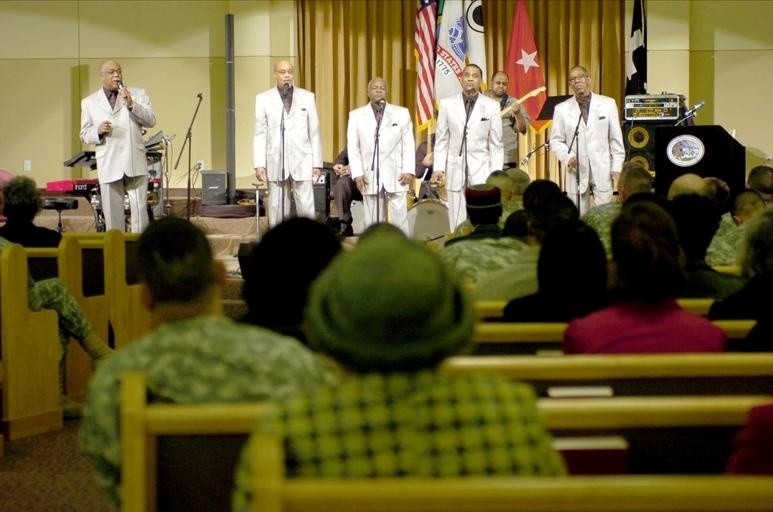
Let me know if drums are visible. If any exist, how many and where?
[407,197,450,240]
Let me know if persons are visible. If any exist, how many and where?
[78,216,566,511]
[331,144,363,239]
[482,72,531,173]
[2,174,62,283]
[547,64,628,217]
[415,134,435,201]
[78,58,155,235]
[728,403,771,475]
[432,64,503,234]
[346,77,415,239]
[251,60,322,234]
[0,238,123,421]
[444,164,772,354]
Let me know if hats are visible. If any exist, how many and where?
[308,220,473,364]
[462,183,508,220]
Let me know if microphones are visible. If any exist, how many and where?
[685,102,704,116]
[283,83,289,98]
[117,82,127,99]
[521,152,531,166]
[466,88,475,103]
[378,100,385,119]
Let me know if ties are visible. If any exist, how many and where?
[108,91,119,118]
[465,99,475,121]
[581,97,591,122]
[375,104,384,125]
[282,90,292,114]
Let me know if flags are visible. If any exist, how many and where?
[430,1,464,117]
[501,0,553,133]
[412,1,436,137]
[462,0,488,88]
[620,1,645,109]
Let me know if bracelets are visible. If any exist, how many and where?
[612,191,619,196]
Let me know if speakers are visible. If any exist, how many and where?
[201,169,228,205]
[291,172,330,222]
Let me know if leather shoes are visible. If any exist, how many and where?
[337,221,351,237]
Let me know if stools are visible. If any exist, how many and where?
[39,196,79,233]
